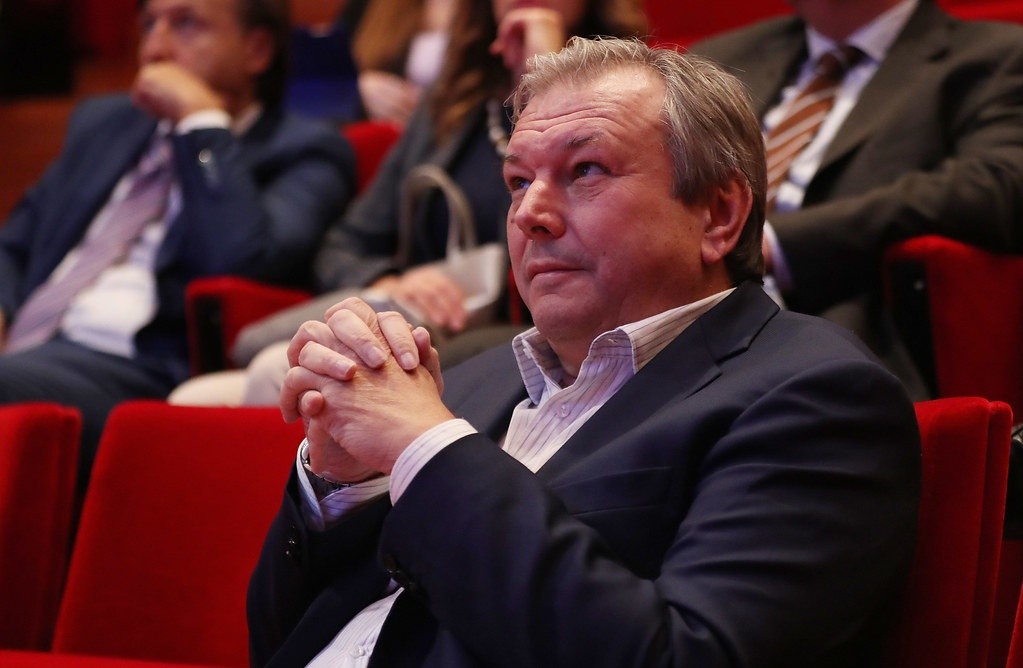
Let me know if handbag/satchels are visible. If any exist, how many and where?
[229,163,503,363]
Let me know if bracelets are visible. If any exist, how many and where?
[299,440,385,497]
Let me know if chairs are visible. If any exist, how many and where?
[0,0,1023,668]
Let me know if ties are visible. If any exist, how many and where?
[5,133,180,355]
[762,48,841,215]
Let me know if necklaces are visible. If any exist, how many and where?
[486,98,509,155]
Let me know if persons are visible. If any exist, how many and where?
[680,0,1023,399]
[248,37,923,668]
[0,0,646,558]
